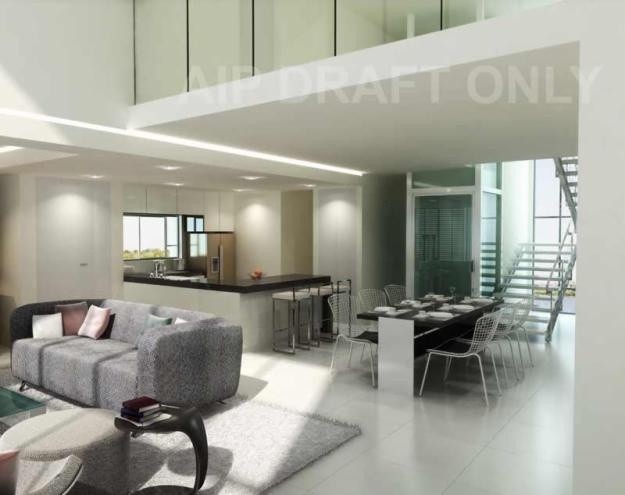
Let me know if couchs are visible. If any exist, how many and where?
[9,299,242,418]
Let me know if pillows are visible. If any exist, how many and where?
[31,300,113,340]
[132,313,186,348]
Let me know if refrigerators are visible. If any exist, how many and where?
[185,231,235,281]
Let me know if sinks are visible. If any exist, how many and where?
[164,276,200,281]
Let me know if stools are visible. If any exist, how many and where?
[1,408,130,494]
[272,279,352,355]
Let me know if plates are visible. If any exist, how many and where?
[375,293,494,322]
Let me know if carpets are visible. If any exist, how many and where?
[0,365,363,494]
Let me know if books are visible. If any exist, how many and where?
[120,395,161,423]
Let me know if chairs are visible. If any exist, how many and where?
[326,284,413,387]
[418,294,535,410]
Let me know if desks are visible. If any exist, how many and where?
[115,402,208,495]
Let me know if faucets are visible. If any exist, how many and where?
[155,262,165,277]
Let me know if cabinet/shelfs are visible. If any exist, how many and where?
[186,188,234,233]
[122,183,204,217]
